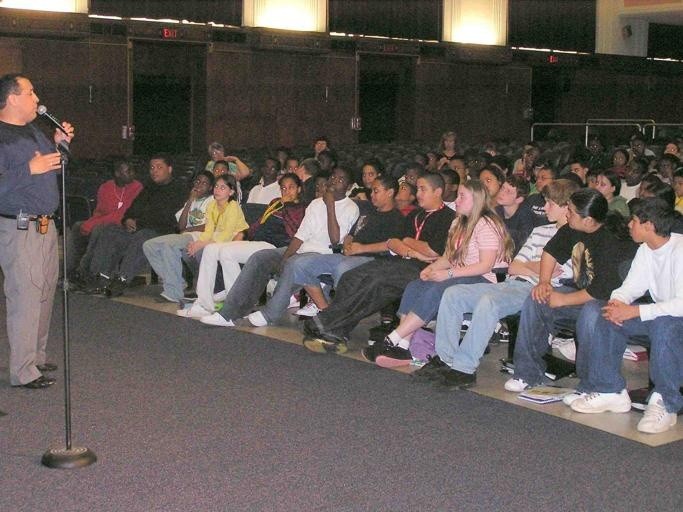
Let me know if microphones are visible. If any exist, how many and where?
[37,105,70,137]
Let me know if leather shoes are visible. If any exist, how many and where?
[36,364,59,373]
[11,373,55,388]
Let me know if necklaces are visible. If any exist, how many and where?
[111,182,126,211]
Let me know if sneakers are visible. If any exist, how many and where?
[212,290,227,302]
[408,354,451,383]
[460,319,472,332]
[636,391,677,435]
[375,344,413,368]
[562,391,585,406]
[247,310,280,326]
[570,386,632,414]
[287,313,320,339]
[151,294,178,303]
[499,330,510,342]
[176,299,212,318]
[200,312,236,327]
[504,377,532,392]
[361,335,394,362]
[294,301,322,317]
[287,294,301,308]
[547,333,576,361]
[70,274,110,295]
[304,333,347,354]
[433,369,476,391]
[105,278,125,298]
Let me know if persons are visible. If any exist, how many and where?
[515,144,570,165]
[426,151,441,173]
[435,131,459,160]
[505,190,638,406]
[612,149,630,166]
[293,174,404,310]
[142,170,214,302]
[349,159,386,198]
[362,179,515,367]
[303,171,454,355]
[414,152,428,171]
[652,153,679,184]
[318,151,336,175]
[182,174,249,302]
[0,72,74,390]
[87,151,191,297]
[628,176,661,216]
[246,158,283,205]
[594,170,630,217]
[199,165,359,327]
[490,155,513,179]
[590,139,605,167]
[314,137,327,159]
[663,141,683,163]
[570,159,592,187]
[480,165,505,220]
[671,169,683,215]
[586,165,606,189]
[559,172,582,187]
[212,156,250,181]
[448,155,470,185]
[494,176,535,259]
[438,170,460,212]
[410,180,580,390]
[284,157,300,174]
[631,138,652,161]
[626,131,655,158]
[475,153,491,175]
[508,166,560,257]
[619,157,649,205]
[405,163,425,188]
[275,146,291,176]
[643,182,675,210]
[204,142,237,181]
[59,159,144,292]
[571,193,683,433]
[296,158,321,204]
[188,172,306,317]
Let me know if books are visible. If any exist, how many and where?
[517,385,577,404]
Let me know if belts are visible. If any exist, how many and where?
[0,212,54,221]
[506,273,528,283]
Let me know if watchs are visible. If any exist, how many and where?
[402,247,411,261]
[447,268,455,279]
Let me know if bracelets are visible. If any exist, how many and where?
[235,159,240,164]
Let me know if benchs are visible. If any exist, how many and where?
[256,272,338,323]
[499,307,683,417]
